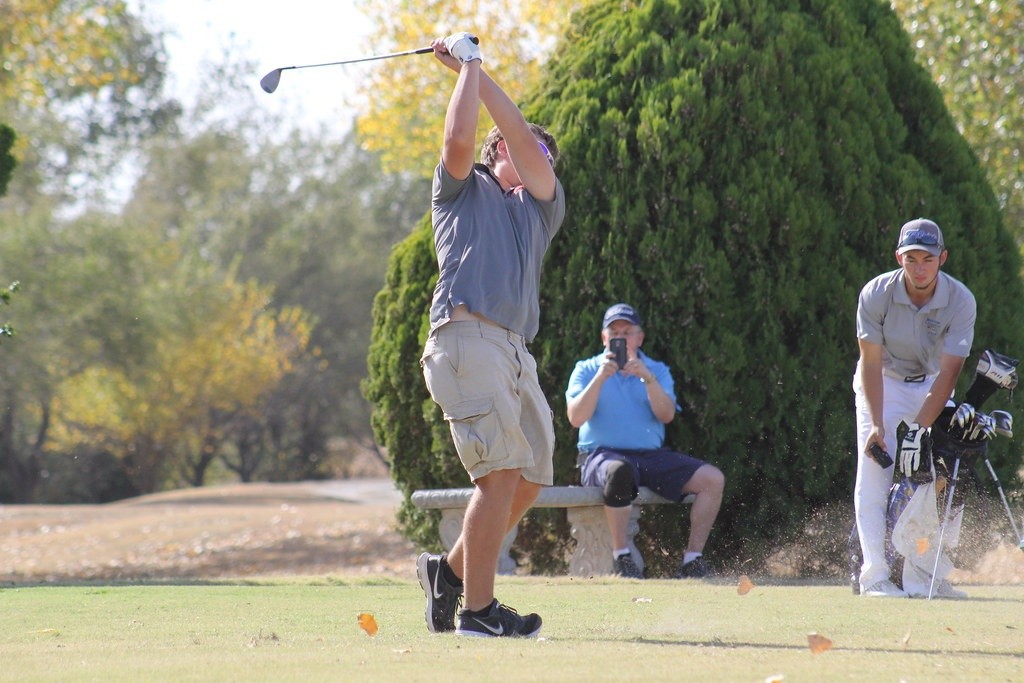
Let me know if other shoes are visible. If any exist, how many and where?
[936,580,968,600]
[865,579,909,598]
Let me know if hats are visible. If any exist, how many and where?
[603,304,640,328]
[896,218,943,256]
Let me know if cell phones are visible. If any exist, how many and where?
[610,338,628,370]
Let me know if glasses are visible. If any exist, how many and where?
[896,233,943,251]
[498,137,554,168]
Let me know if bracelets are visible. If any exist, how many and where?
[639,373,657,384]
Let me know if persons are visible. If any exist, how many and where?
[564,304,726,580]
[852,217,976,597]
[415,34,566,639]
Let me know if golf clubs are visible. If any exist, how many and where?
[945,399,1014,441]
[260,36,481,94]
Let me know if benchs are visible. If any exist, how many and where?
[410,487,697,578]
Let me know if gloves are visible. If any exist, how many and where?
[899,422,930,478]
[443,31,482,66]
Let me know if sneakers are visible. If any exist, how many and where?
[454,598,543,638]
[613,552,645,579]
[416,551,464,634]
[680,555,712,577]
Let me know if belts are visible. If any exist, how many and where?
[884,368,939,383]
